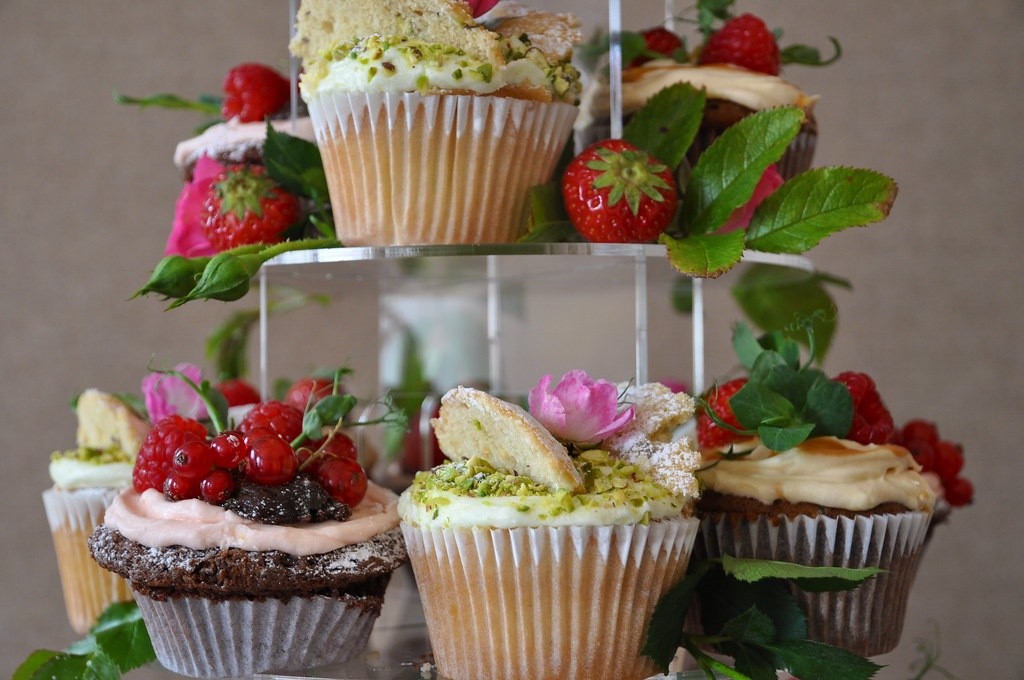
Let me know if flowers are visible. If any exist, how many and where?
[164,151,227,259]
[528,368,635,456]
[141,361,211,424]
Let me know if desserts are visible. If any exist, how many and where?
[46,0,948,680]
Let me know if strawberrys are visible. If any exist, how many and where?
[202,163,299,254]
[563,139,675,242]
